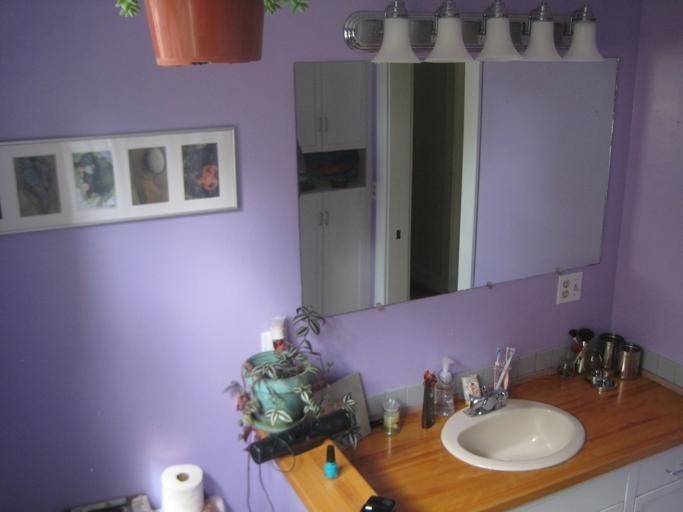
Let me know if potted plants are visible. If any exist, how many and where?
[113,0,310,66]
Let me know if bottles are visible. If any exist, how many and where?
[420,371,435,426]
[322,444,336,477]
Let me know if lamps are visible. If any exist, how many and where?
[343,0,605,63]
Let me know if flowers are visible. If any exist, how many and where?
[223,306,361,452]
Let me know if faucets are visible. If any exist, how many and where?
[482,388,509,411]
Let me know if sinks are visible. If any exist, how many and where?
[439,398,587,472]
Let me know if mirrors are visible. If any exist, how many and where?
[295,59,621,318]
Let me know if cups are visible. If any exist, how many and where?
[492,366,513,396]
[382,399,400,435]
[557,351,617,392]
[598,332,642,380]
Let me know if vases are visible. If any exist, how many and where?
[242,352,309,432]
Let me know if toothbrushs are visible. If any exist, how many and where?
[493,346,517,390]
[503,346,509,391]
[494,346,502,385]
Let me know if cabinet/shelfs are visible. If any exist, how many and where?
[297,62,371,154]
[299,187,374,317]
[507,445,683,512]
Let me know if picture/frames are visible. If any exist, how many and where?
[312,371,372,452]
[0,128,237,230]
